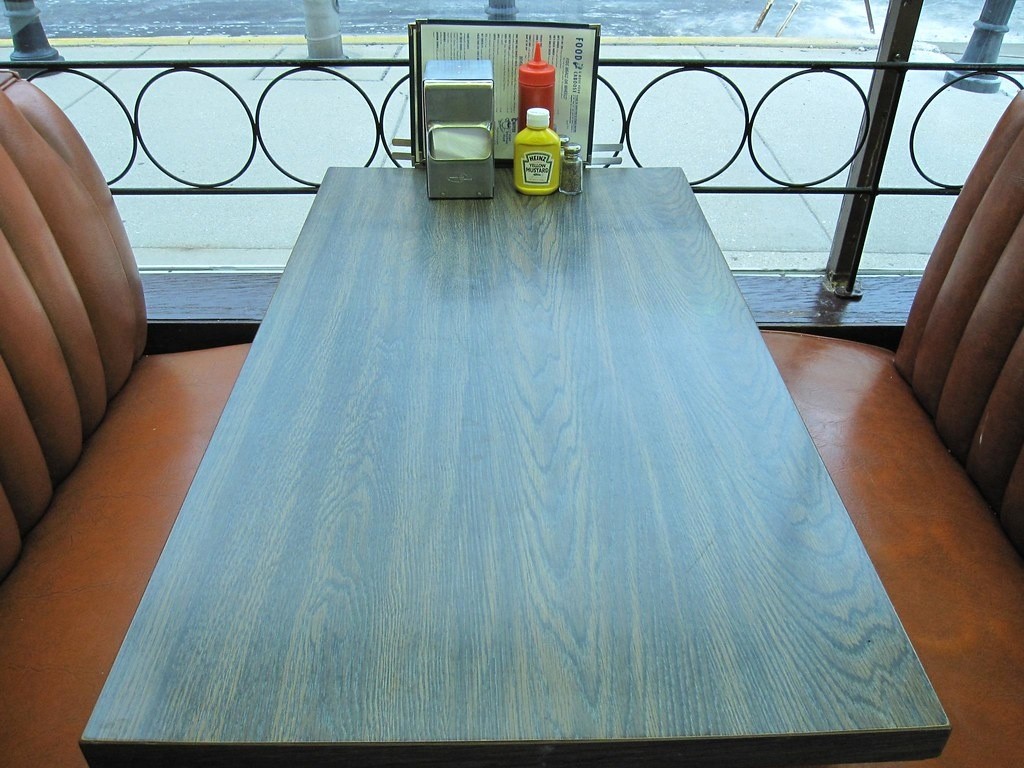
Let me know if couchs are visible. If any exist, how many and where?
[0,68,256,768]
[754,81,1024,768]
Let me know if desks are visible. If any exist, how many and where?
[79,163,953,768]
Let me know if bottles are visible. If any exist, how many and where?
[518,42,555,131]
[559,143,582,194]
[559,135,570,150]
[514,108,561,195]
